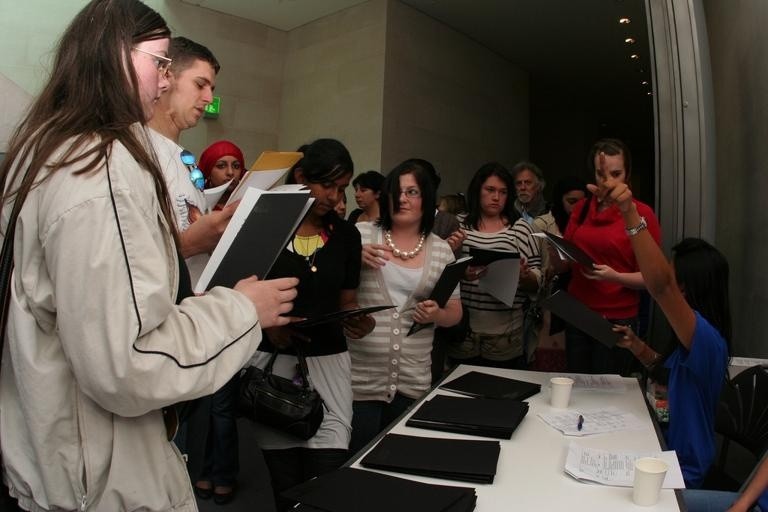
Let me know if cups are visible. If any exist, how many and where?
[632,457,667,506]
[545,378,576,407]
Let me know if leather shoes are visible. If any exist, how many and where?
[195,480,214,500]
[213,485,236,504]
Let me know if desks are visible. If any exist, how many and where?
[294,361,687,512]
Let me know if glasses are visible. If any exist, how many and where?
[130,44,174,76]
[400,189,423,199]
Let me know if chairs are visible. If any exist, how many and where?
[701,359,767,490]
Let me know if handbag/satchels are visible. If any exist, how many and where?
[236,331,326,441]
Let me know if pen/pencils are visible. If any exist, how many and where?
[578,415,583,432]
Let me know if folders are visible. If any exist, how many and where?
[462,243,522,267]
[404,367,541,439]
[406,255,473,338]
[204,168,317,293]
[541,227,602,273]
[296,433,501,511]
[263,305,396,335]
